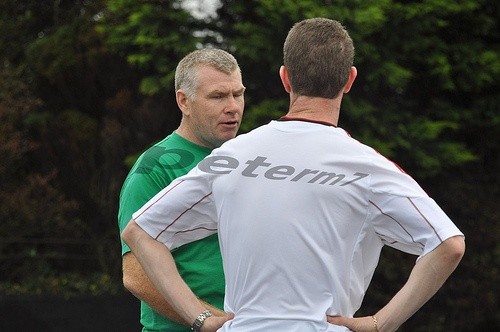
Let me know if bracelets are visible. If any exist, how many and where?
[191,310,212,332]
[373,314,379,332]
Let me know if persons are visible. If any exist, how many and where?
[119,48,245,332]
[120,17,465,332]
